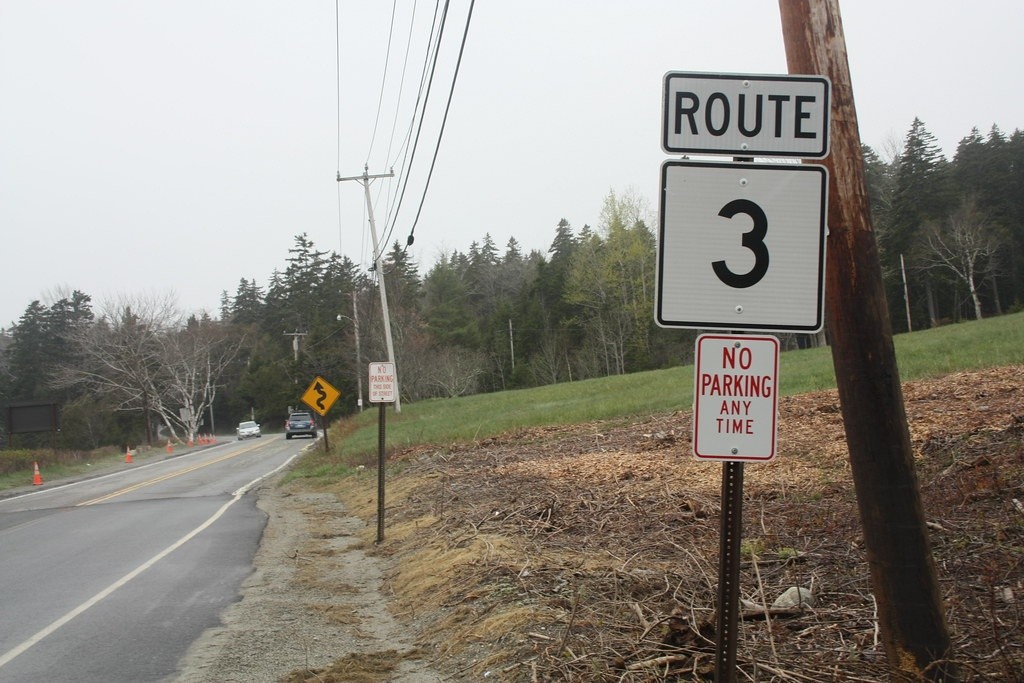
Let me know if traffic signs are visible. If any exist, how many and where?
[368,361,395,404]
[691,334,780,463]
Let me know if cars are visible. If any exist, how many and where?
[284,411,316,439]
[235,419,261,440]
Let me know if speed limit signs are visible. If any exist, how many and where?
[653,157,831,335]
